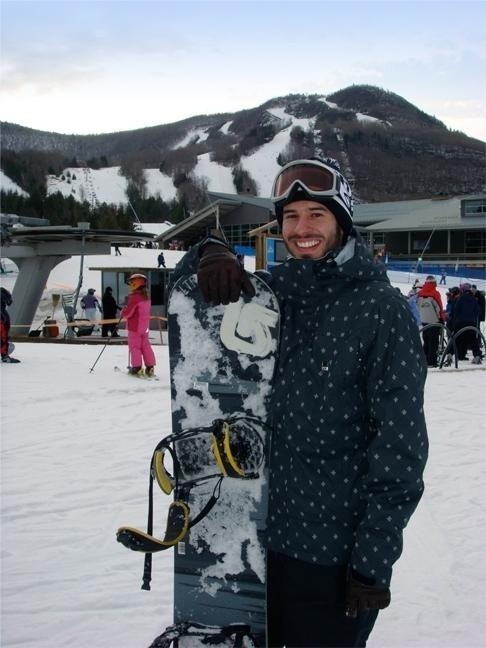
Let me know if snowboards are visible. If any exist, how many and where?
[167,270,283,648]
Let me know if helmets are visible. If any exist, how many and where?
[127,274,147,290]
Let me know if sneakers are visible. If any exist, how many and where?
[130,367,142,376]
[146,366,153,376]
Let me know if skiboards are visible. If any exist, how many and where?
[114,365,158,381]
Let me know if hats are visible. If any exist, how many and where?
[274,156,353,232]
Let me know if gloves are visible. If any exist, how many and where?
[198,242,255,304]
[344,584,390,617]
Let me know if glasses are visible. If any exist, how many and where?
[269,159,349,204]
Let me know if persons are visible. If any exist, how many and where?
[100,285,121,337]
[79,287,101,331]
[113,242,121,257]
[0,287,20,362]
[117,271,157,379]
[157,250,166,268]
[396,267,485,369]
[165,154,429,648]
